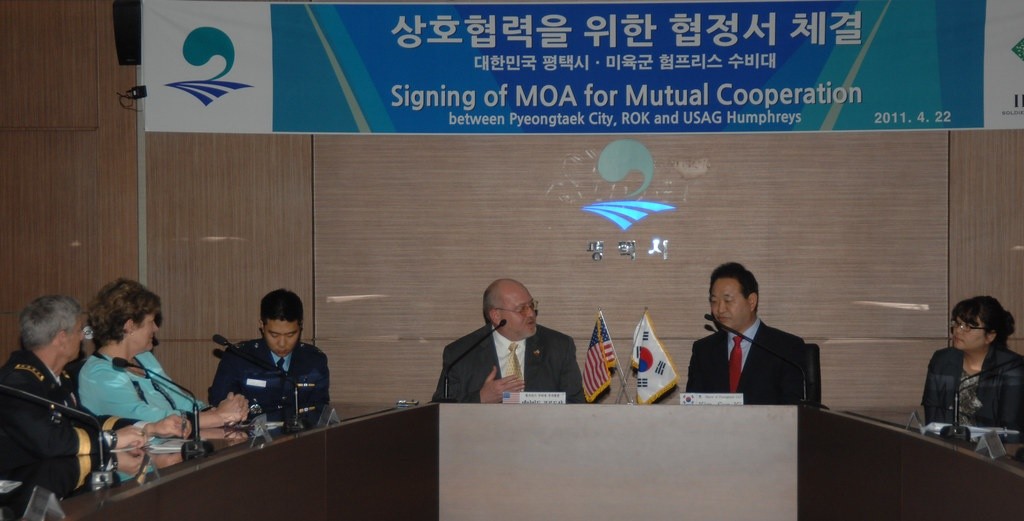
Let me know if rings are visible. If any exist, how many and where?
[139,429,145,436]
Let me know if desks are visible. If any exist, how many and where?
[0,403,1024,521]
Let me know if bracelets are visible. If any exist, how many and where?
[104,429,117,449]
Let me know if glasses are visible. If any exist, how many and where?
[490,301,539,317]
[949,317,988,333]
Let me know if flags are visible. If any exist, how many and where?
[581,312,619,403]
[630,310,680,405]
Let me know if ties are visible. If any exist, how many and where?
[277,357,287,380]
[728,334,744,393]
[65,394,75,408]
[506,341,522,384]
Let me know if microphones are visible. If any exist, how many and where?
[112,357,213,453]
[704,314,807,400]
[435,318,507,402]
[212,334,310,432]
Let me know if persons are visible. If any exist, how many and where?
[921,296,1024,420]
[431,279,587,403]
[79,279,250,427]
[686,262,811,405]
[0,449,144,521]
[209,288,330,407]
[0,293,192,473]
